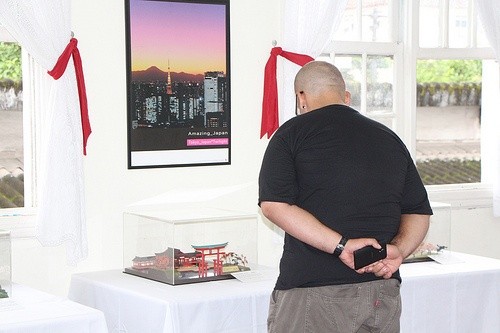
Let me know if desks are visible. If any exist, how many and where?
[69,249,500,333]
[0,281,109,333]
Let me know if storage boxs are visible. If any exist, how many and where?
[404,200,453,263]
[0,228,12,300]
[121,198,259,287]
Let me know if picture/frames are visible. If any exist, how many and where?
[125,0,232,170]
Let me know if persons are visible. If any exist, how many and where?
[345,81,393,109]
[417,81,479,107]
[256,60,434,333]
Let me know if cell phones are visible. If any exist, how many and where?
[354,241,389,270]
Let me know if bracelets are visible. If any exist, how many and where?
[333,235,351,259]
